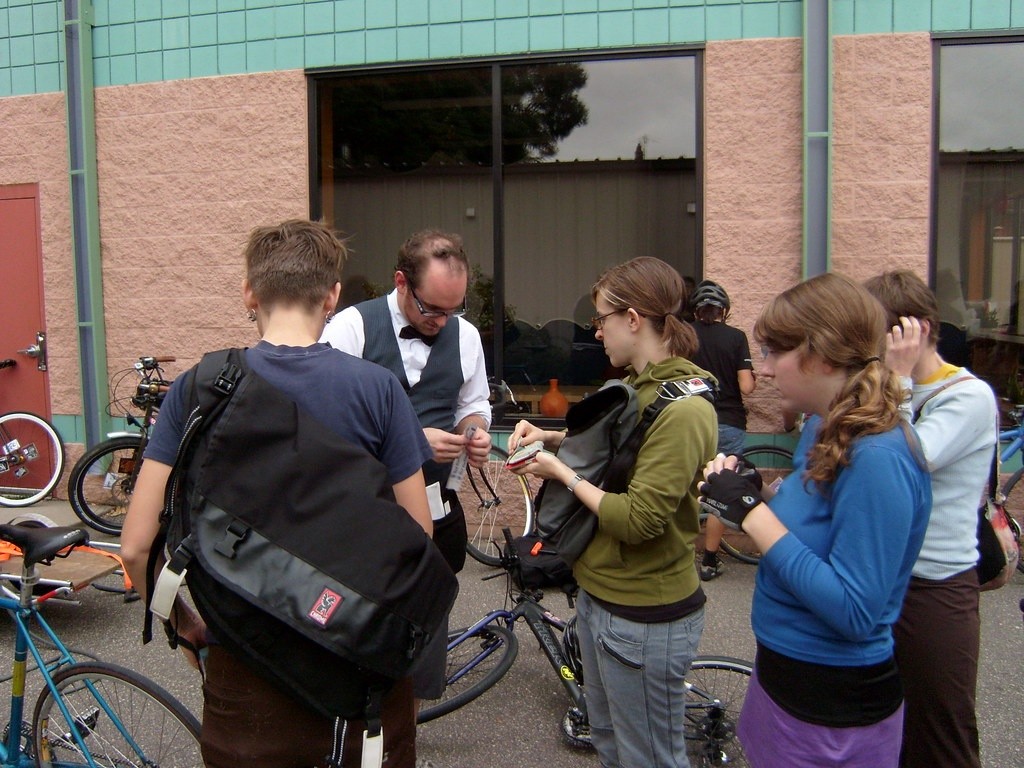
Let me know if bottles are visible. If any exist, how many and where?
[541,379,569,418]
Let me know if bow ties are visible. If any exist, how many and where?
[399,325,439,346]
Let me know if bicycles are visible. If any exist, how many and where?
[718,443,794,566]
[449,374,537,568]
[999,396,1024,576]
[375,522,758,768]
[67,353,177,537]
[0,519,209,768]
[0,359,67,508]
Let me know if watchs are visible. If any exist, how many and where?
[567,474,585,493]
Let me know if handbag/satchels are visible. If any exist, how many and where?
[158,345,460,720]
[977,495,1018,592]
[532,378,715,567]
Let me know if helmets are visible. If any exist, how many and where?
[690,279,730,314]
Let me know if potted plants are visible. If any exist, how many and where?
[1005,368,1024,429]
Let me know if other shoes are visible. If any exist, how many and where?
[699,564,725,579]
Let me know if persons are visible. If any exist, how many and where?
[506,252,721,768]
[119,214,435,768]
[697,271,933,768]
[316,225,496,736]
[684,279,756,581]
[859,271,999,768]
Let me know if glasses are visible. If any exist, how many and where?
[591,309,645,330]
[406,279,466,319]
